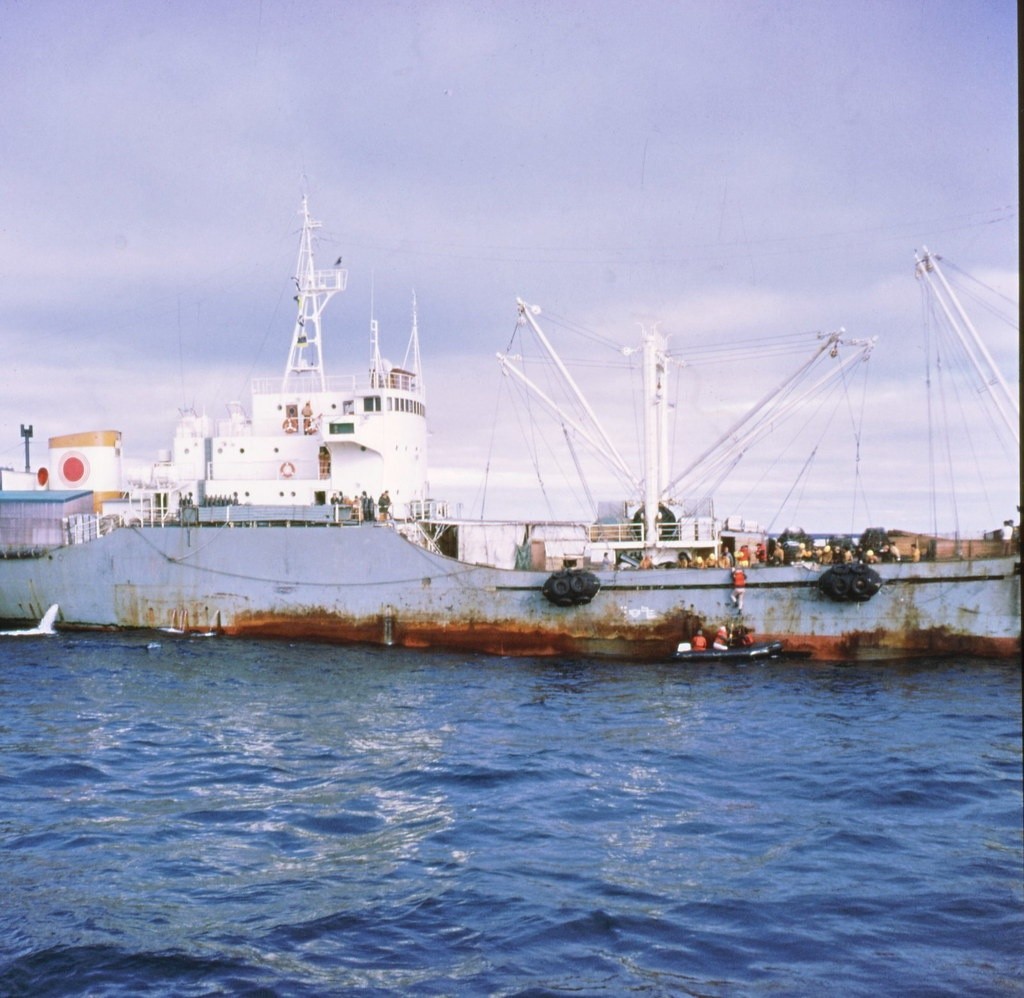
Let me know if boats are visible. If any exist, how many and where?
[0,194,1020,659]
[667,640,786,662]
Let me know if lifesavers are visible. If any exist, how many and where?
[283,419,298,434]
[551,569,591,607]
[281,462,295,478]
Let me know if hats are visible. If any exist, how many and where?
[844,550,852,557]
[709,553,714,560]
[776,542,782,548]
[720,626,726,631]
[804,551,812,557]
[696,556,702,564]
[697,630,703,635]
[799,543,806,549]
[824,545,831,553]
[866,550,874,557]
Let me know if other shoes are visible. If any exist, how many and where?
[737,609,741,614]
[733,602,738,607]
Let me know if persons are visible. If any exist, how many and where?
[330,490,391,522]
[730,566,747,615]
[733,623,756,648]
[690,629,707,651]
[302,402,312,435]
[677,542,785,569]
[796,539,921,567]
[602,552,613,571]
[713,624,732,651]
[1000,521,1014,557]
